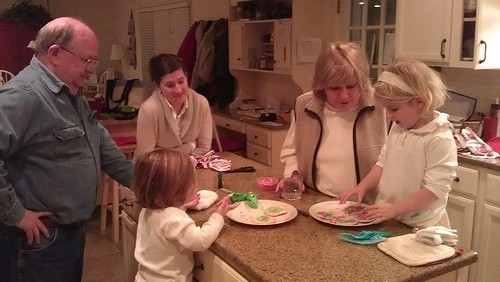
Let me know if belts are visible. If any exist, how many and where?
[40,219,86,229]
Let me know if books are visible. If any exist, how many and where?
[243,48,256,68]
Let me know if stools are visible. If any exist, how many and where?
[98,135,136,244]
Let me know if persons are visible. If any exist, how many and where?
[275,41,388,198]
[134,147,241,282]
[0,16,136,282]
[136,53,212,157]
[338,58,458,229]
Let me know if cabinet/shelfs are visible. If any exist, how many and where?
[119,184,249,282]
[395,0,499,65]
[212,110,291,179]
[229,17,297,74]
[440,166,500,281]
[0,19,37,77]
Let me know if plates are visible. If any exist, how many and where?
[309,201,380,227]
[225,199,298,225]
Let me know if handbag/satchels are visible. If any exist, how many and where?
[106,77,143,113]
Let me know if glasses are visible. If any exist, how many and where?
[47,44,101,67]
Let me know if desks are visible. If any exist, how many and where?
[90,100,139,208]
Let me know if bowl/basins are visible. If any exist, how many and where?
[259,113,277,122]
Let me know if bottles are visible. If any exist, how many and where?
[260,56,266,69]
